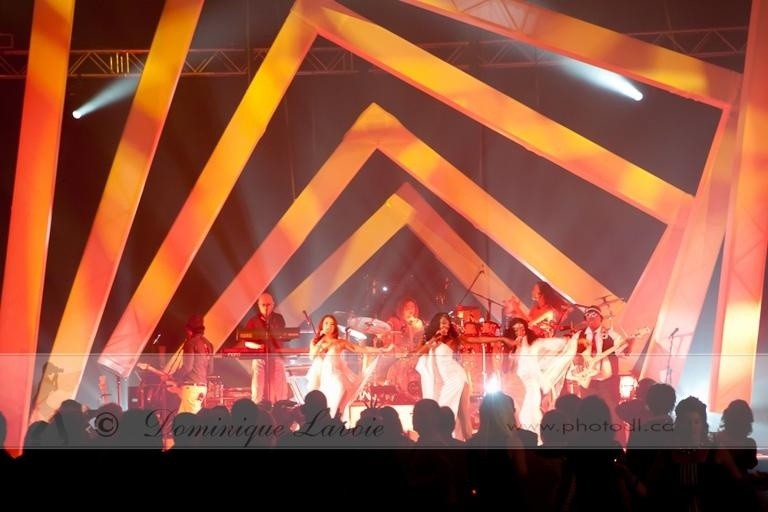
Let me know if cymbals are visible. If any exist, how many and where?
[349,317,390,334]
[597,294,625,307]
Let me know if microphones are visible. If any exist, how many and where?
[512,337,522,353]
[433,328,446,338]
[152,334,161,345]
[318,328,329,341]
[479,318,484,329]
[302,310,310,322]
[669,328,678,337]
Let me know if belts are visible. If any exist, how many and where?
[184,382,205,386]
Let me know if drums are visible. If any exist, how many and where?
[361,338,384,351]
[480,320,499,337]
[387,355,423,404]
[385,331,403,345]
[457,306,482,320]
[462,321,479,336]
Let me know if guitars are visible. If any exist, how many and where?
[136,362,187,397]
[565,326,653,388]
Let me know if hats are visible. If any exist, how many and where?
[584,305,603,319]
[185,313,204,328]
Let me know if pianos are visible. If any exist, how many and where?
[236,327,300,341]
[222,346,308,360]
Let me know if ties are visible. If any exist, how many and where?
[590,333,597,358]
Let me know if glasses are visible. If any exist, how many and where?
[583,312,597,318]
